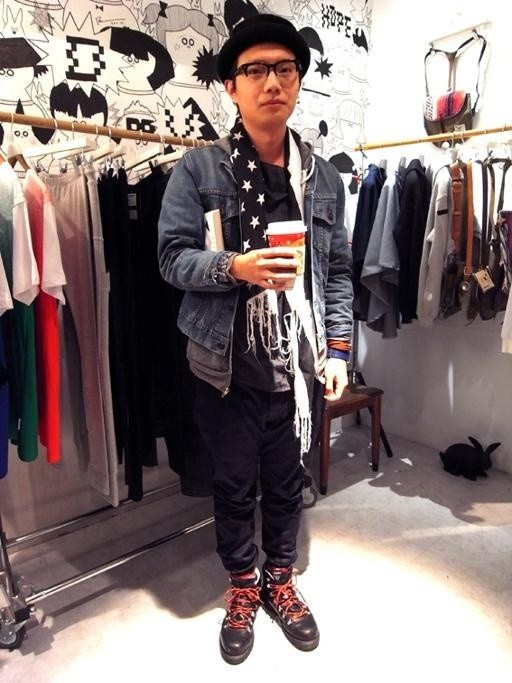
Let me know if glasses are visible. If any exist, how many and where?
[231,57,302,78]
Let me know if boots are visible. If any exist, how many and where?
[219,561,321,665]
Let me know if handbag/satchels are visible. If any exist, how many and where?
[439,210,512,321]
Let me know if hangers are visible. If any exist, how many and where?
[351,123,512,182]
[2,114,215,182]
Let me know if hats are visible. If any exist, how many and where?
[216,14,310,84]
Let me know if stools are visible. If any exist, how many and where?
[320,384,384,494]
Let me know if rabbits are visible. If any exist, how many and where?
[439,436,501,481]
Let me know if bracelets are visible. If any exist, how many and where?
[328,349,349,362]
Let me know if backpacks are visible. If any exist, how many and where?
[423,91,473,148]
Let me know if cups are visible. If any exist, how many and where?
[265,221,308,276]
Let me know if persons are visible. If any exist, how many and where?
[157,13,354,664]
